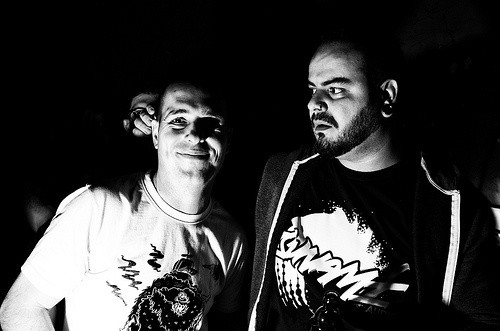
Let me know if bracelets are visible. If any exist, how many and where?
[130,107,144,118]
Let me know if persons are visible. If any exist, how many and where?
[128,39,499,330]
[0,81,252,331]
